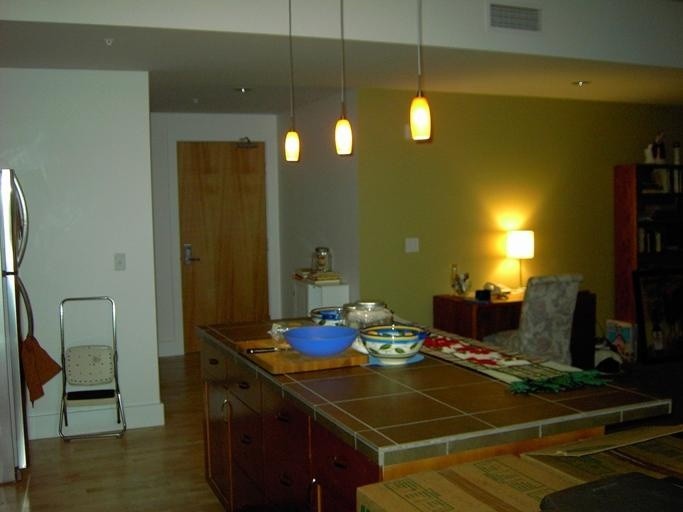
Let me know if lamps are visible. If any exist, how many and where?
[506,230,534,292]
[284,1,300,161]
[409,1,431,143]
[335,3,352,156]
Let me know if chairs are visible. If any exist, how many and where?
[57,295,126,441]
[482,271,583,368]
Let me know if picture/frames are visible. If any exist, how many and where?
[631,271,683,363]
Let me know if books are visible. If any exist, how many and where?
[603,317,639,366]
[636,202,682,254]
[290,267,343,287]
[639,167,682,195]
[635,262,681,276]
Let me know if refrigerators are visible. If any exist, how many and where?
[0,167,34,487]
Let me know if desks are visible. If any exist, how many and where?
[432,290,595,371]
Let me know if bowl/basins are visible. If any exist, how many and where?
[310,306,345,326]
[360,324,427,359]
[282,325,358,356]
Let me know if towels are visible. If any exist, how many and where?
[20,334,62,408]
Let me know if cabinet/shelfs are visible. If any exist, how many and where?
[292,278,349,318]
[263,380,379,512]
[197,328,232,512]
[613,163,682,364]
[232,350,262,512]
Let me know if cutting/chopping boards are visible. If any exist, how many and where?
[235,337,368,376]
